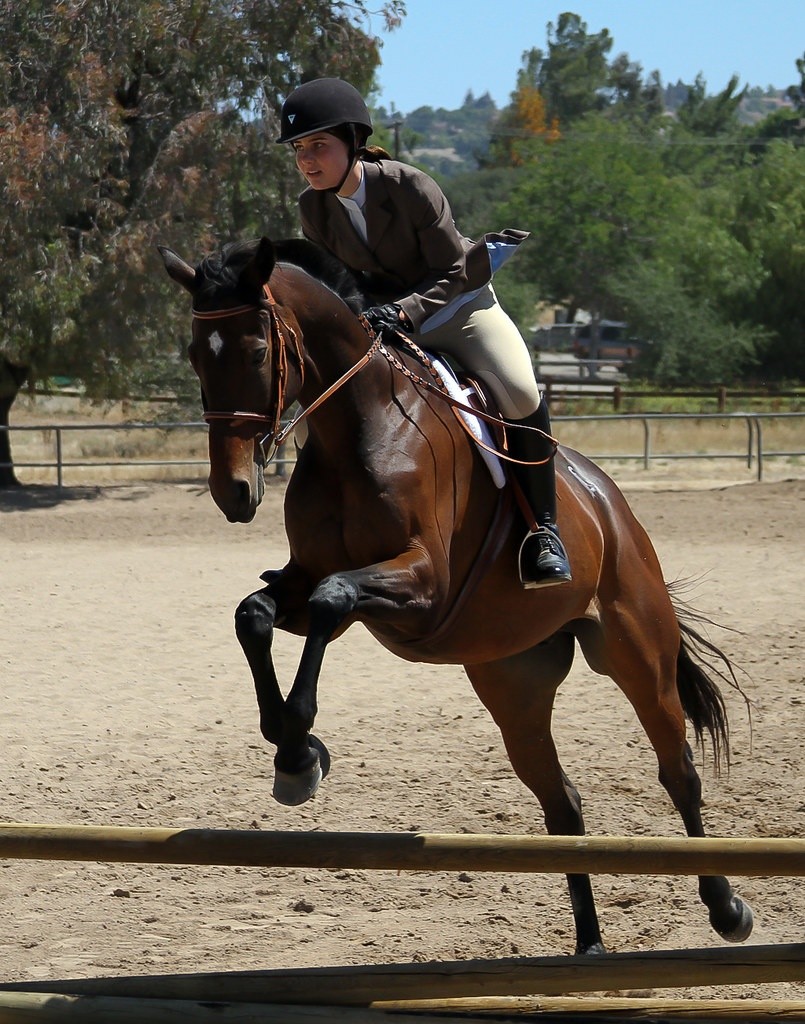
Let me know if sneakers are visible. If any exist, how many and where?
[258,567,284,581]
[513,521,574,588]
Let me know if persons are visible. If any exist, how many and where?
[275,78,573,592]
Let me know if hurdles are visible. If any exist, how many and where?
[0,823,805,1024]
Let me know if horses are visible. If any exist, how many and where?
[148,233,762,967]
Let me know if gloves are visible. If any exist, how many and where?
[364,301,404,336]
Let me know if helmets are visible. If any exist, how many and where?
[279,75,375,142]
